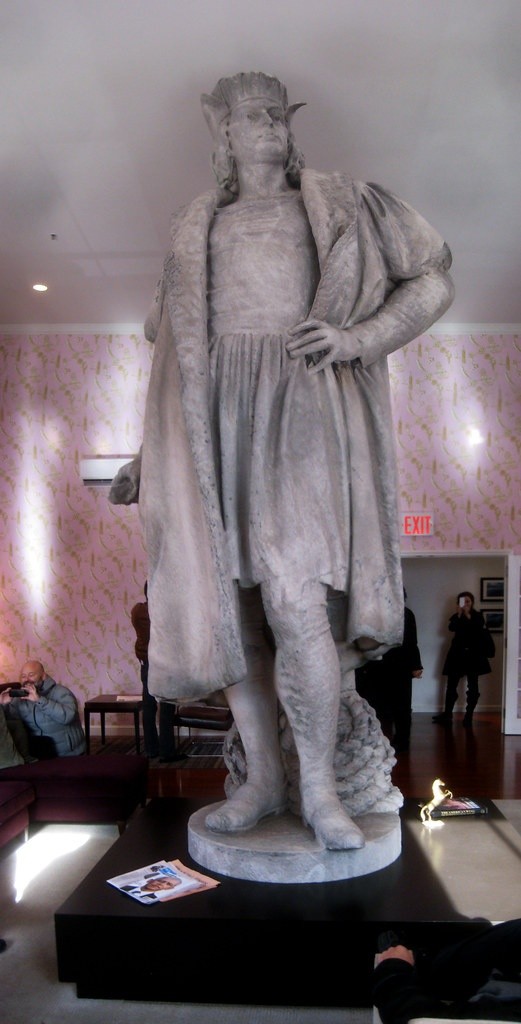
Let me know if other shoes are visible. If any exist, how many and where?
[159,748,178,760]
[148,748,160,758]
[390,734,410,751]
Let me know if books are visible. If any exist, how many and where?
[417,796,489,818]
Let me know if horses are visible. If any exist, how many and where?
[417,777,453,822]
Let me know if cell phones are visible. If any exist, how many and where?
[9,689,25,697]
[459,597,465,607]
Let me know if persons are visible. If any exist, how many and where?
[433,592,496,723]
[121,875,182,900]
[370,919,521,1024]
[111,72,456,849]
[0,661,86,763]
[131,580,176,764]
[357,586,423,749]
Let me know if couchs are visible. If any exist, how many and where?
[0,682,149,834]
[0,781,35,850]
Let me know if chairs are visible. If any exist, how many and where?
[171,690,234,758]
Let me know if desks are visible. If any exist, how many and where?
[84,695,144,756]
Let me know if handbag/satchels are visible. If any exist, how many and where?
[482,623,495,658]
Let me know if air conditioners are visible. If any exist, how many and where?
[79,458,134,486]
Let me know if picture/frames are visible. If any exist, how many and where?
[479,577,504,603]
[480,608,503,633]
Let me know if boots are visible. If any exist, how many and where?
[432,690,458,720]
[463,693,480,727]
